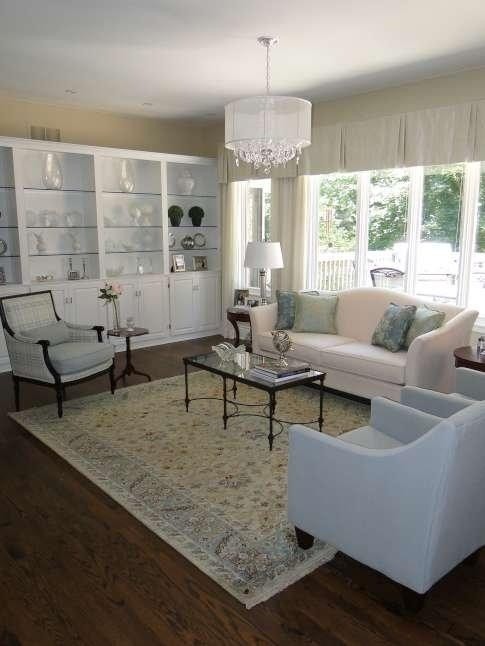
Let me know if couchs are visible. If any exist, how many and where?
[249,287,479,402]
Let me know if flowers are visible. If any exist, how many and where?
[98,280,125,305]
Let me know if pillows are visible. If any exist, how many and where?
[371,303,416,353]
[275,289,319,330]
[404,303,446,348]
[292,292,338,334]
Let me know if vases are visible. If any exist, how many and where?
[110,300,123,332]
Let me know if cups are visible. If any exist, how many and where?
[237,352,249,371]
[125,318,135,331]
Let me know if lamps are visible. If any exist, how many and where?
[223,36,312,176]
[244,240,285,306]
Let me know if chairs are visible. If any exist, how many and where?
[0,290,116,418]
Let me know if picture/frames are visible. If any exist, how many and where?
[233,289,249,306]
[171,255,187,272]
[192,256,208,271]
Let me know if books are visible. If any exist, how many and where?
[250,356,311,384]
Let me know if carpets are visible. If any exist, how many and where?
[8,370,370,611]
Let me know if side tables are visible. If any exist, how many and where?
[227,305,252,353]
[454,346,485,372]
[107,327,151,389]
